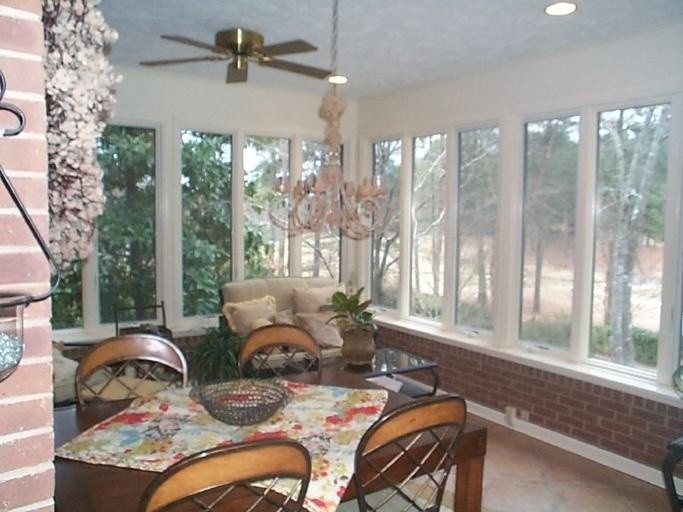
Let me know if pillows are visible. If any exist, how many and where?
[223,297,277,333]
[293,284,349,315]
[312,324,343,348]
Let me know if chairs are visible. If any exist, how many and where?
[73,334,187,432]
[354,392,467,511]
[110,298,171,342]
[139,439,312,511]
[239,325,321,384]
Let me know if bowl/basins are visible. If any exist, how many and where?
[189,377,294,427]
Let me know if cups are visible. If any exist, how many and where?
[397,350,407,368]
[375,350,385,368]
[385,350,395,369]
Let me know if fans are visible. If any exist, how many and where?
[137,24,335,90]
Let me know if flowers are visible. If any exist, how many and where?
[319,287,375,330]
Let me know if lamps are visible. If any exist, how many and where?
[268,0,389,243]
[0,68,62,387]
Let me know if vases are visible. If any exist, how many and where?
[337,322,377,367]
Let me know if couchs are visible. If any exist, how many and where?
[218,275,348,372]
